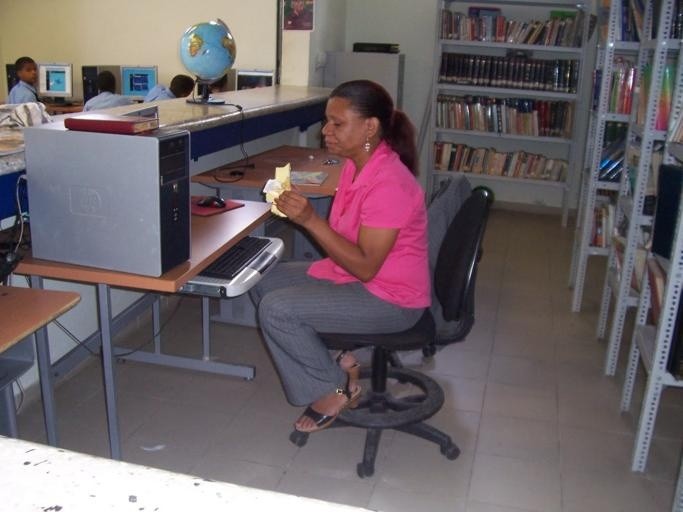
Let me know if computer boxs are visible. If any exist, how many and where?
[6,63,19,94]
[82,65,121,105]
[198,69,236,95]
[22,121,192,277]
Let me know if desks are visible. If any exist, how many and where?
[1,145,352,461]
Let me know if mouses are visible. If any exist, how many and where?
[196,195,226,208]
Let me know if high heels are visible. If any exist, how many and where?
[295,372,363,434]
[334,349,360,381]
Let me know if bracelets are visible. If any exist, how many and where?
[320,159,340,166]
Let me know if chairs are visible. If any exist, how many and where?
[287,171,493,479]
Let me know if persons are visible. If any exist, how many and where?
[82,70,134,113]
[143,74,195,104]
[7,56,40,104]
[250,78,432,434]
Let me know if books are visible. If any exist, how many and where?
[0,130,26,158]
[433,5,588,181]
[63,113,160,134]
[289,169,329,186]
[584,1,683,385]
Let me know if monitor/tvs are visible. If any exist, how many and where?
[122,105,159,129]
[122,65,159,103]
[38,64,73,106]
[236,72,274,90]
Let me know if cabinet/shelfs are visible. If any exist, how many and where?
[424,1,683,475]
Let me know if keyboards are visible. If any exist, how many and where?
[198,236,272,279]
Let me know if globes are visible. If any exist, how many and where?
[177,19,238,105]
